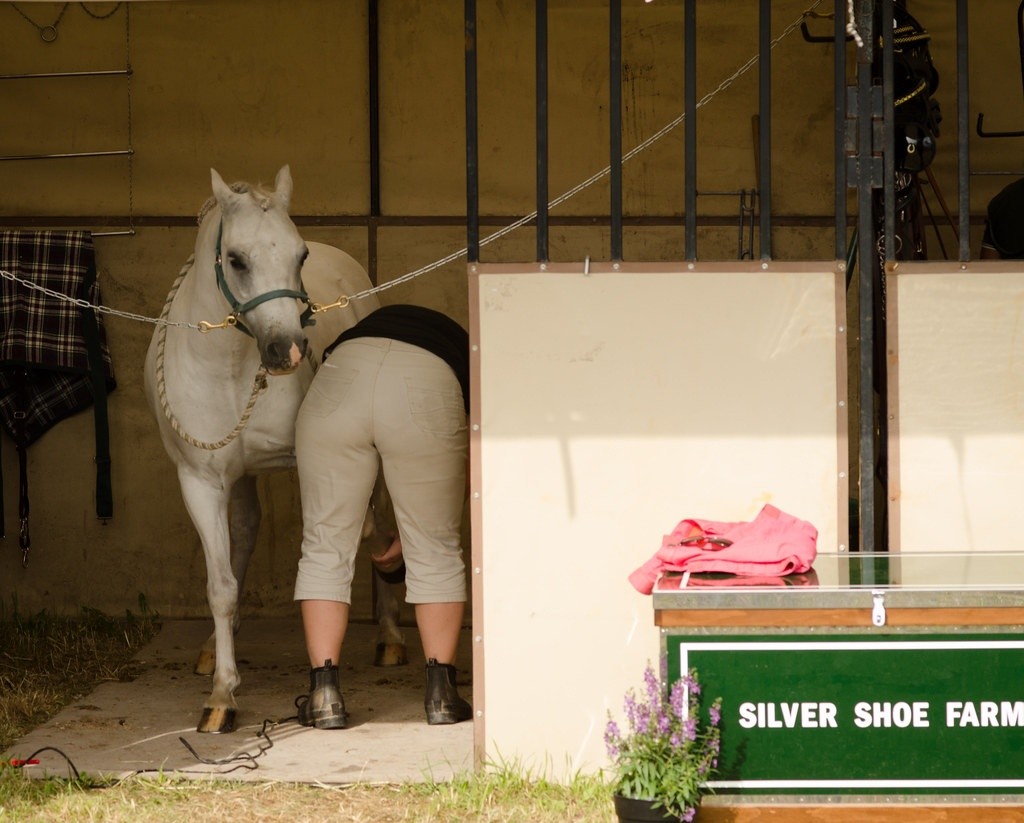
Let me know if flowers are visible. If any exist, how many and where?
[605,660,724,823]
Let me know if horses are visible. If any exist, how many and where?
[144,162,407,733]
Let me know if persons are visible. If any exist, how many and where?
[291,302,473,732]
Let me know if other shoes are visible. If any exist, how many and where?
[297,659,346,729]
[423,657,474,726]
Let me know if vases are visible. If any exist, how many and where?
[614,793,702,823]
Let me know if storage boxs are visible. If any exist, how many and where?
[650,550,1024,808]
